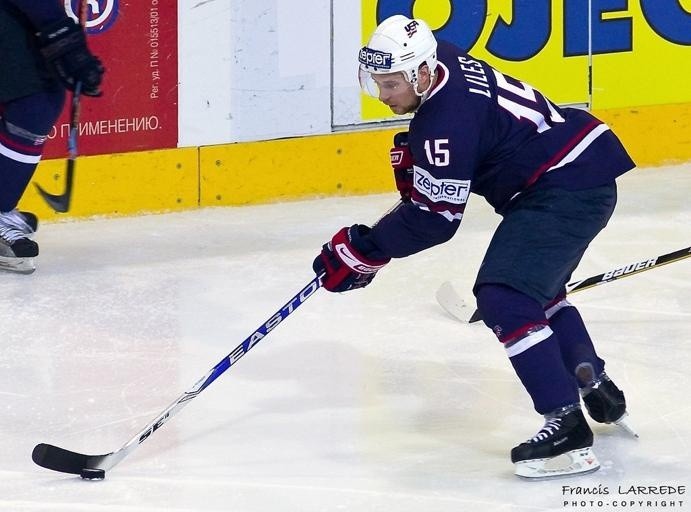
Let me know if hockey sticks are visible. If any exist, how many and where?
[437,247,690,323]
[32,200,404,474]
[34,80,81,213]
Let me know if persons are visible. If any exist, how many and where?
[0,0,106,258]
[313,14,637,464]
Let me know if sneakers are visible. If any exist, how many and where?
[2,210,39,234]
[585,376,627,424]
[0,224,39,259]
[510,402,593,461]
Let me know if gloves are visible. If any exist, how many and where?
[311,224,390,293]
[33,16,106,99]
[388,131,415,201]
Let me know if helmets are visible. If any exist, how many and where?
[358,14,440,87]
